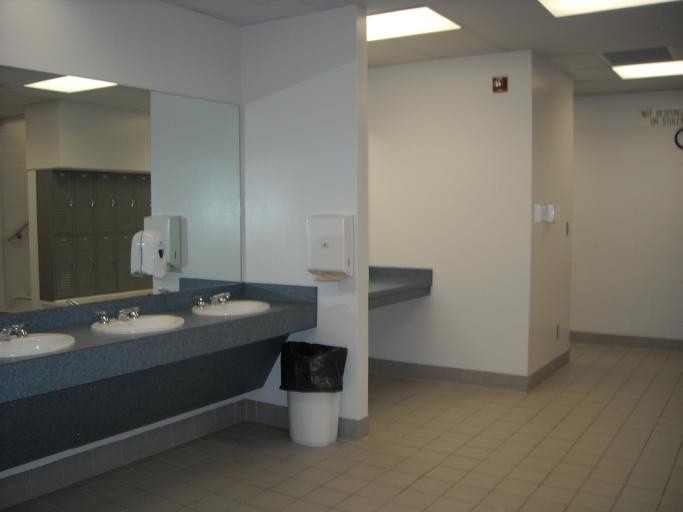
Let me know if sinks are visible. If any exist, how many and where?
[0,333,76,360]
[191,300,271,316]
[90,314,185,335]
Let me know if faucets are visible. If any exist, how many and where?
[12,321,29,337]
[210,291,231,305]
[115,307,141,321]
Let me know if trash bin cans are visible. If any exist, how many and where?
[280,341,347,447]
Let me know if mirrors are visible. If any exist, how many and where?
[0,65,243,312]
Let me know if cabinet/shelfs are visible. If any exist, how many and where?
[36,170,153,301]
